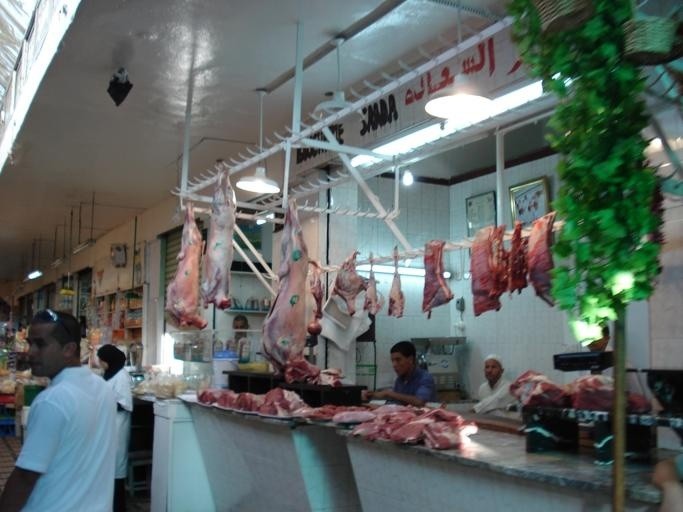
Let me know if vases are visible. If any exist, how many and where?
[465,191,497,258]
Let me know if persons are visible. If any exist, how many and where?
[651,460,683,512]
[470,353,517,415]
[1,308,118,512]
[95,343,134,512]
[361,341,437,407]
[571,325,665,414]
[225,315,249,350]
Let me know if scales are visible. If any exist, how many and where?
[554,353,615,375]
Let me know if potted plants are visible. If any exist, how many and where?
[506,0,663,348]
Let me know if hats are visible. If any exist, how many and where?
[484,354,504,371]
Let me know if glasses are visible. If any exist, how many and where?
[35,307,72,340]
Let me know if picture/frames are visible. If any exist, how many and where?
[509,175,550,228]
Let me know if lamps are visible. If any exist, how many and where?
[402,167,414,186]
[351,72,573,168]
[312,36,365,124]
[424,0,495,119]
[356,264,453,280]
[236,88,281,194]
[28,192,96,281]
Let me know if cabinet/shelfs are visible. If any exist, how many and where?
[91,282,148,368]
